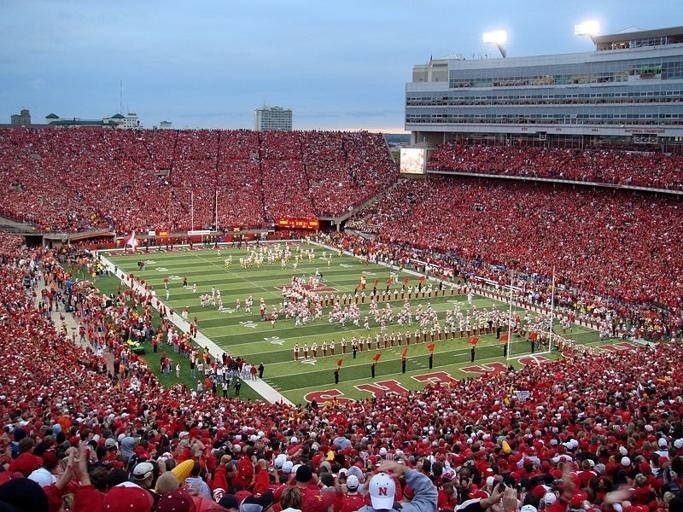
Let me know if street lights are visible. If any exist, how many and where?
[575,17,601,51]
[482,30,510,59]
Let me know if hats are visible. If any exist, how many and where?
[0,386,273,512]
[418,406,683,512]
[273,423,404,512]
